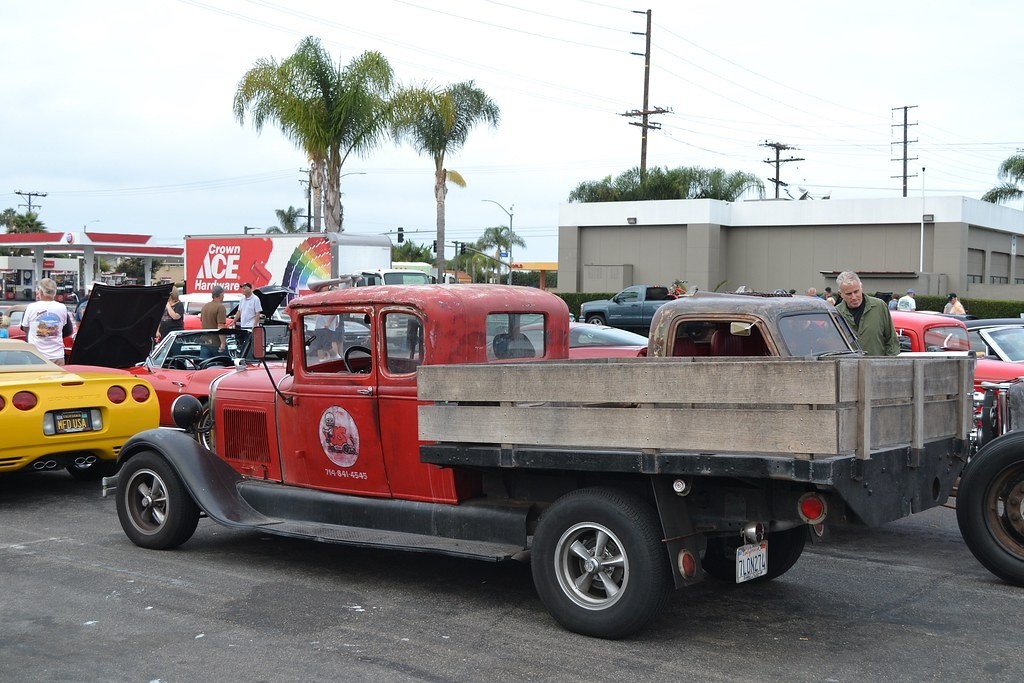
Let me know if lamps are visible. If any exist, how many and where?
[923,215,934,221]
[627,218,636,223]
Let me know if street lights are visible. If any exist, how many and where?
[482,199,513,286]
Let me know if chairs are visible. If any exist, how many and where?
[710,329,744,356]
[673,337,696,356]
[493,334,536,359]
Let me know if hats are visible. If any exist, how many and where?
[906,288,914,293]
[240,283,252,289]
[86,281,99,291]
[947,293,957,300]
[211,286,223,297]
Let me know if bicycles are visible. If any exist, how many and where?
[663,281,687,300]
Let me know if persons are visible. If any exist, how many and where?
[889,289,916,312]
[944,293,966,314]
[229,283,263,333]
[786,288,837,353]
[315,312,345,362]
[200,286,229,357]
[0,277,108,367]
[155,286,184,358]
[835,271,900,356]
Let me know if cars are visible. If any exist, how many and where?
[829,306,1024,474]
[4,305,80,343]
[174,285,646,366]
[62,282,290,456]
[0,338,161,488]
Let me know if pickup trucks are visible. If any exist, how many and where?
[102,287,978,640]
[578,287,675,337]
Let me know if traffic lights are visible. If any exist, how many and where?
[461,243,466,254]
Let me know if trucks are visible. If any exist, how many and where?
[184,233,436,310]
[391,261,460,284]
